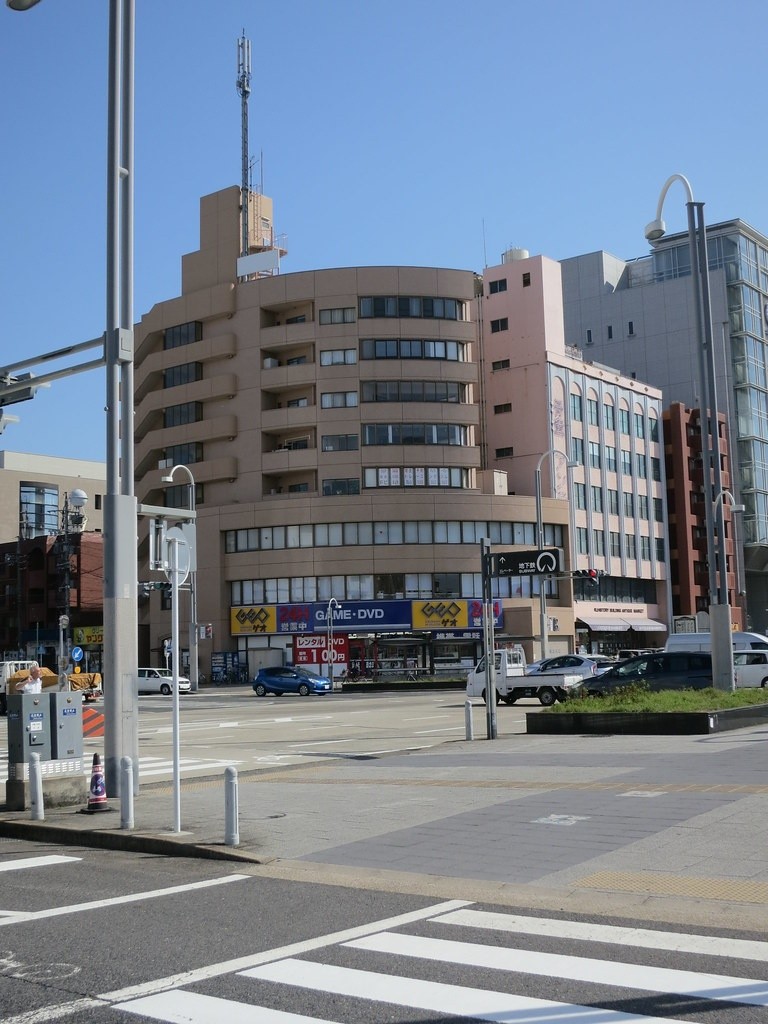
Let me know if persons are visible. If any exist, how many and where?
[15,666,42,694]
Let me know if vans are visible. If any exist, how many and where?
[567,652,737,700]
[665,633,768,652]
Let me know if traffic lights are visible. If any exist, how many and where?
[575,570,600,579]
[154,583,173,591]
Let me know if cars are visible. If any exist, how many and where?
[526,654,625,680]
[252,666,332,697]
[732,650,768,687]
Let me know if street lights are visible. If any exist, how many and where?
[644,175,737,693]
[534,449,580,659]
[162,465,198,691]
[327,599,342,692]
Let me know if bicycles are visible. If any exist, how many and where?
[235,666,249,686]
[215,667,233,687]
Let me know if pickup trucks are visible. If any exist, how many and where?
[0,661,100,713]
[466,647,583,706]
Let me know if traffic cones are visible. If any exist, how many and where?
[76,753,118,815]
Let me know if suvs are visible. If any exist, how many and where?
[138,668,191,695]
